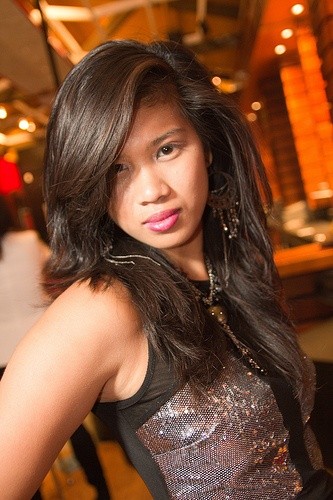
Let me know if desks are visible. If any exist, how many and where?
[271,243,333,329]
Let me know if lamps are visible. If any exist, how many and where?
[0,0,304,184]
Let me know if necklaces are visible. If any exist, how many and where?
[151,247,263,372]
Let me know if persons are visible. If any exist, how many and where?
[0,35,333,500]
[0,192,58,370]
